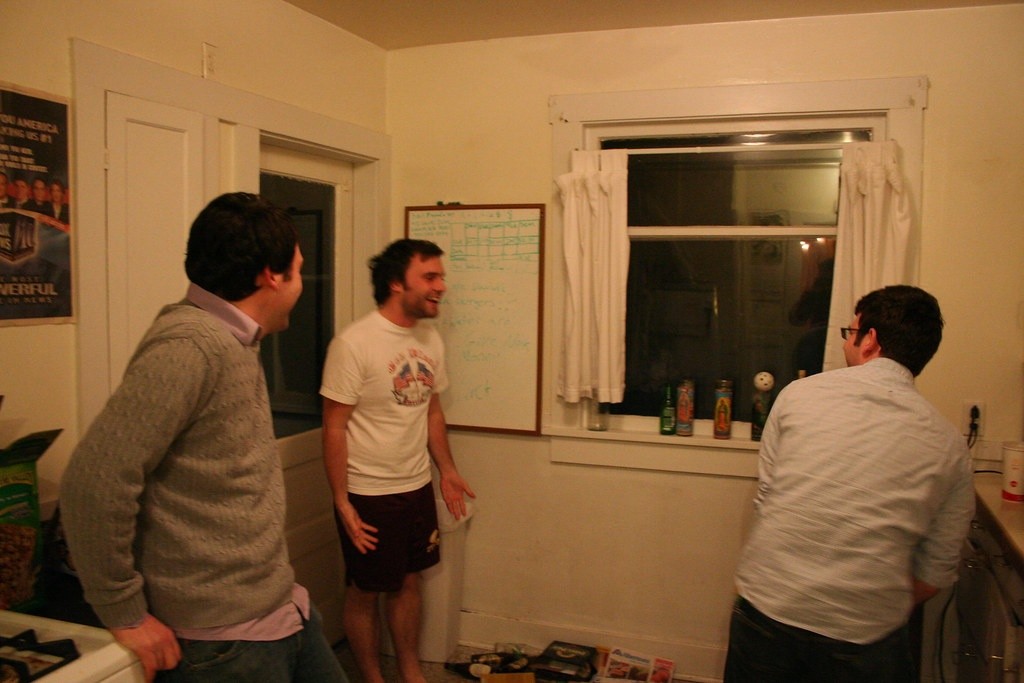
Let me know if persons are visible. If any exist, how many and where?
[721,283,975,681]
[320,236,476,683]
[59,192,349,683]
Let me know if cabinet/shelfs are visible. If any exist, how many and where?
[954,474,1024,683]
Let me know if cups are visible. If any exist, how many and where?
[1000,440,1024,502]
[586,400,609,429]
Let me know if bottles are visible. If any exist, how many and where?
[676,378,695,435]
[797,369,807,379]
[713,379,733,439]
[660,384,677,434]
[751,388,776,441]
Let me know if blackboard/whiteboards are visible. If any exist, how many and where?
[403,203,546,435]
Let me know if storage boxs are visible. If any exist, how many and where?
[0,395,66,609]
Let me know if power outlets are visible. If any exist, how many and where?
[962,399,985,437]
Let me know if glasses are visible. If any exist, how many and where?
[840,327,859,340]
